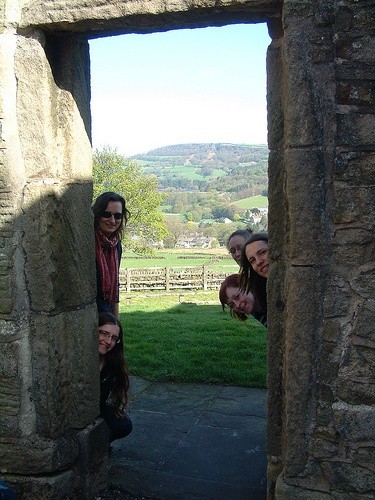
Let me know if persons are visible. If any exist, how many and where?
[239,233,269,295]
[226,228,253,267]
[92,192,131,321]
[98,312,133,457]
[219,274,267,327]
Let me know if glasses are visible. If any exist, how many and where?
[101,211,123,219]
[98,329,120,343]
